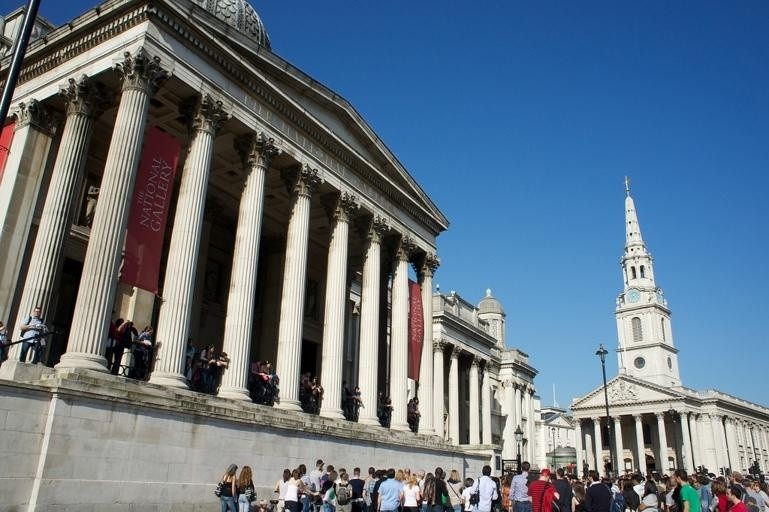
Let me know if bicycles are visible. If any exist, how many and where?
[262,489,319,512]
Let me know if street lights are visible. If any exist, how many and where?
[595,342,616,481]
[551,426,556,470]
[747,425,757,462]
[668,405,680,472]
[513,424,524,473]
[721,414,732,474]
[686,411,695,475]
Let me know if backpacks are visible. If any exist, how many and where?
[337,483,349,505]
[215,480,225,497]
[244,488,256,502]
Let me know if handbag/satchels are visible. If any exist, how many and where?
[469,494,479,506]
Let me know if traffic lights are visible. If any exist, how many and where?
[755,461,760,472]
[748,468,753,473]
[698,466,701,473]
[754,466,758,474]
[720,468,724,475]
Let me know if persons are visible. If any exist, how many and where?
[407,397,420,432]
[342,380,363,421]
[298,373,323,413]
[380,218,386,226]
[10,51,172,128]
[20,307,46,363]
[247,359,278,406]
[215,449,769,512]
[377,391,392,428]
[208,100,223,118]
[105,310,154,380]
[1,322,8,361]
[265,138,275,150]
[348,195,355,205]
[185,339,230,395]
[310,168,318,179]
